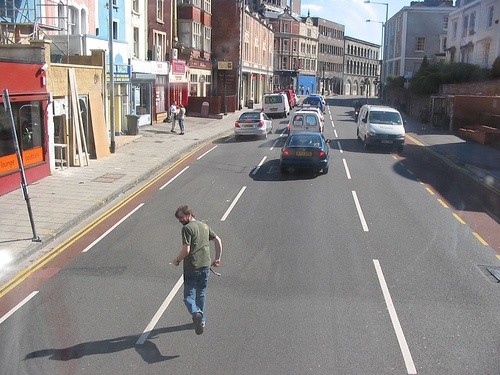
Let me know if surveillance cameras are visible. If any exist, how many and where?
[41,63,48,72]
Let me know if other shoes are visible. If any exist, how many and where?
[179,133,184,135]
[171,130,175,133]
[192,312,205,336]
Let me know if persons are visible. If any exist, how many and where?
[301,85,309,95]
[170,101,185,135]
[173,205,222,335]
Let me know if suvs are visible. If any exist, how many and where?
[287,111,324,136]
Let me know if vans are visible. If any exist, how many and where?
[356,104,407,153]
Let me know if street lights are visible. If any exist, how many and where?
[363,0,389,102]
[365,19,383,99]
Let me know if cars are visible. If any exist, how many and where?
[280,131,332,174]
[261,87,328,121]
[233,111,273,142]
